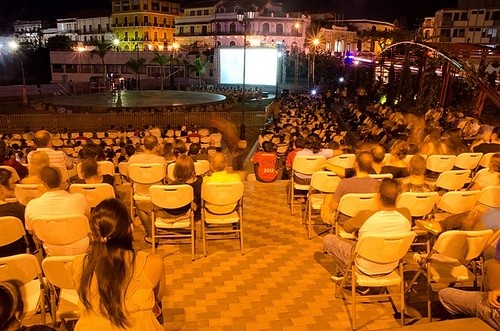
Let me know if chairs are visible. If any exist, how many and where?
[0,88,500,331]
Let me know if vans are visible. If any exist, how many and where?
[88,74,122,92]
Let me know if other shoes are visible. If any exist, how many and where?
[331,271,352,285]
[296,194,305,202]
[351,285,371,294]
[145,235,159,245]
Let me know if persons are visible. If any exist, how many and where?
[293,133,343,203]
[470,127,500,171]
[249,141,279,183]
[0,142,29,179]
[414,210,471,235]
[21,151,50,184]
[27,130,75,178]
[383,141,413,171]
[313,145,397,176]
[71,156,119,197]
[330,151,382,211]
[125,135,168,195]
[0,125,216,173]
[25,166,91,278]
[72,199,166,331]
[437,287,500,331]
[282,139,306,180]
[33,77,264,112]
[468,153,500,192]
[166,141,187,174]
[135,155,203,248]
[202,152,240,235]
[322,176,413,280]
[259,86,484,150]
[399,154,433,228]
[0,169,36,258]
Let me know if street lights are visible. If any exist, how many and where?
[311,38,319,85]
[236,4,256,102]
[167,42,179,90]
[7,40,28,106]
